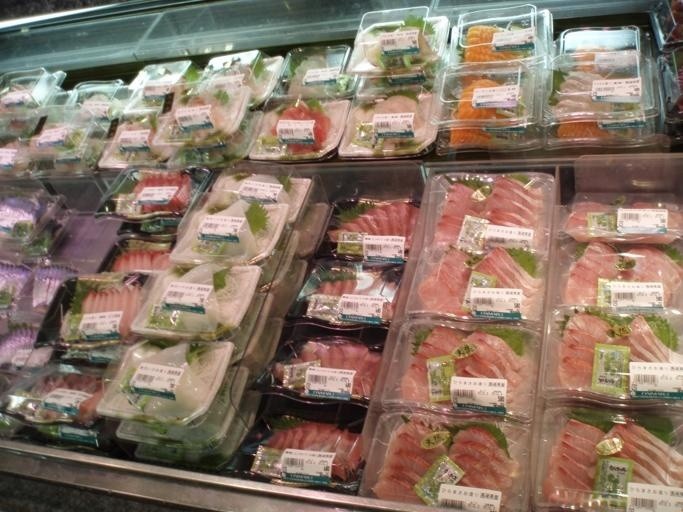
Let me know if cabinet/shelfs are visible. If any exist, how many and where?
[0,0,682,512]
